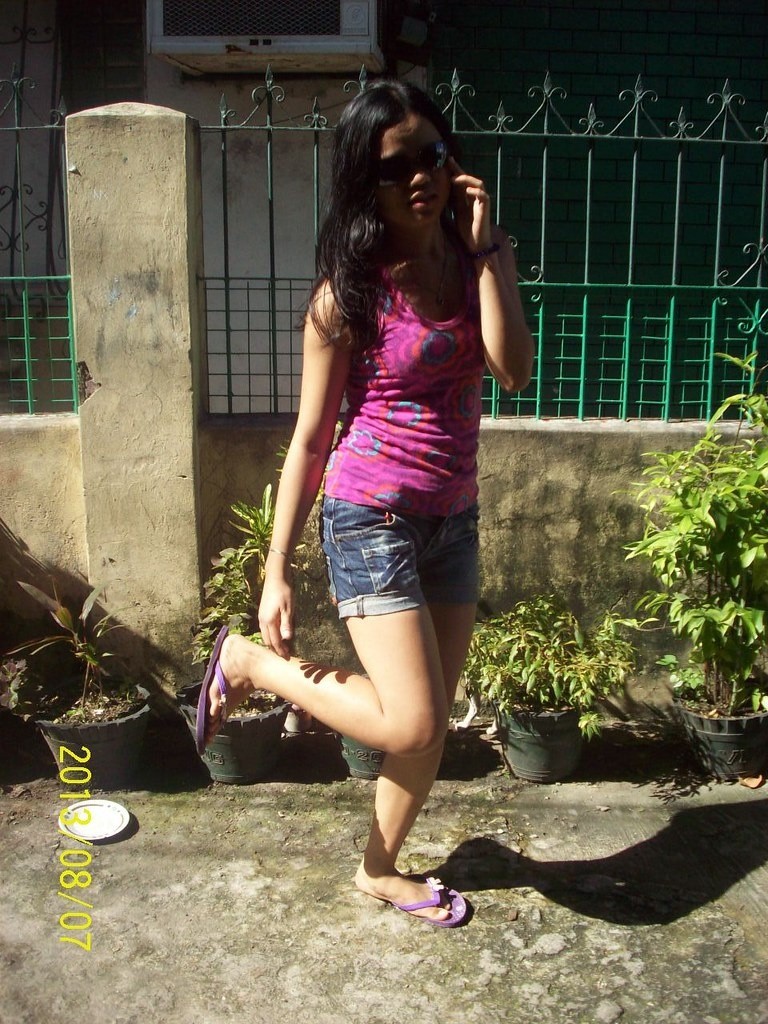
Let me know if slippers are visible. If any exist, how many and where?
[195,625,235,755]
[392,874,470,929]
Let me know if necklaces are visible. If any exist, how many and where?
[413,242,450,306]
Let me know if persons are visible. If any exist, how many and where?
[196,79,534,930]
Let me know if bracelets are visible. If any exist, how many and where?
[470,246,499,268]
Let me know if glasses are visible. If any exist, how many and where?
[379,139,448,187]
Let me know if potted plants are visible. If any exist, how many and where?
[17,576,154,790]
[609,351,768,781]
[173,484,310,787]
[460,592,637,784]
